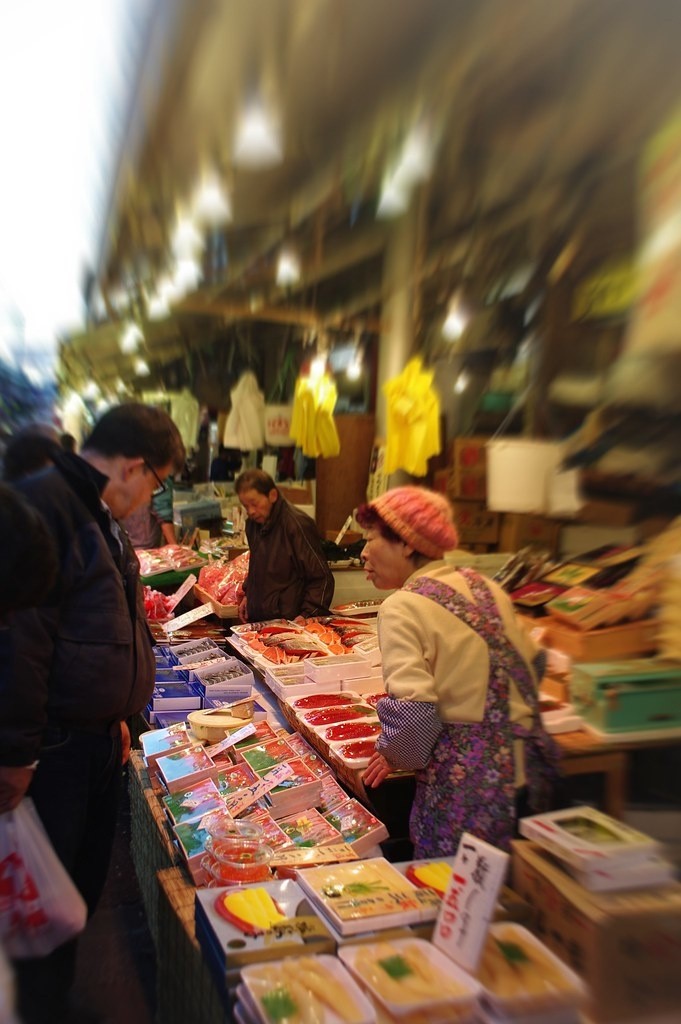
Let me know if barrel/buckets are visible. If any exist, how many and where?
[485,439,546,514]
[540,439,583,518]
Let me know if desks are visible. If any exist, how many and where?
[552,734,681,825]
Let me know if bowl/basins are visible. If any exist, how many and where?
[188,709,252,744]
[201,819,275,887]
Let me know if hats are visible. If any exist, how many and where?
[355,486,457,557]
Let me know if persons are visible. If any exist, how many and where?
[0,401,189,1024]
[232,468,337,625]
[354,484,575,865]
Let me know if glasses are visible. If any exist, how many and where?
[145,459,167,498]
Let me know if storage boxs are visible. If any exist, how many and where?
[436,437,662,561]
[511,805,681,1024]
[508,606,681,731]
[175,499,222,537]
[144,636,390,886]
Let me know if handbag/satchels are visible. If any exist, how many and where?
[0,797,87,959]
